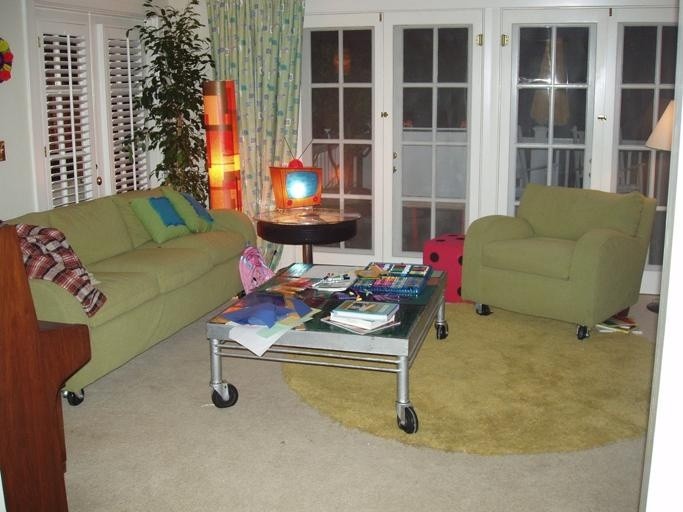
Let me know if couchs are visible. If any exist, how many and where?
[6,185,283,405]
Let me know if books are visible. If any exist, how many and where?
[596,314,643,336]
[210,258,431,339]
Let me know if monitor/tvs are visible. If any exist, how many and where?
[271,167,322,209]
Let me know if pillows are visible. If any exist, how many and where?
[129,194,191,244]
[161,186,215,232]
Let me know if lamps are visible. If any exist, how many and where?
[645,100,674,313]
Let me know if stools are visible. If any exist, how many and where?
[422,234,475,304]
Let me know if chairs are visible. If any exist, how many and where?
[461,183,657,340]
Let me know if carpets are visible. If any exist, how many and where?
[279,302,655,455]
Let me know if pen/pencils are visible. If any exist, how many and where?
[353,262,431,290]
[324,273,350,281]
